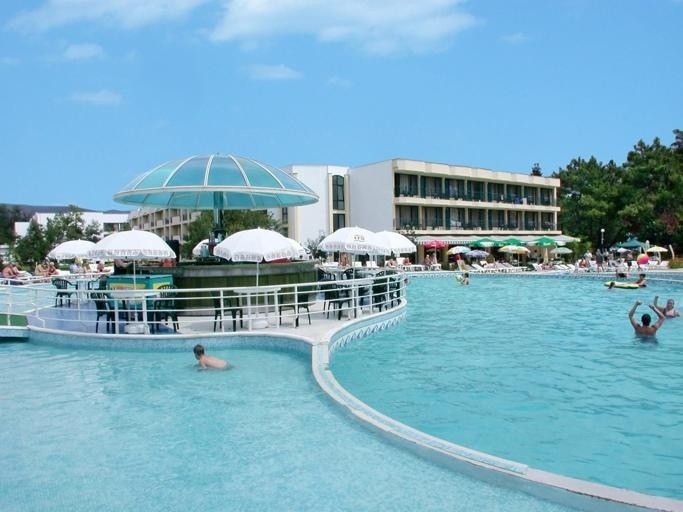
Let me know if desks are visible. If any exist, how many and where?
[233,287,281,331]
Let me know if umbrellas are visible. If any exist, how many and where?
[422,240,449,261]
[317,225,416,279]
[447,235,575,267]
[617,239,644,248]
[213,227,309,318]
[47,228,176,321]
[646,246,668,252]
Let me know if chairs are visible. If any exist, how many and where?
[52,279,78,308]
[279,286,313,326]
[531,259,669,272]
[384,257,442,271]
[209,290,243,331]
[0,263,114,286]
[457,260,527,274]
[91,284,180,334]
[318,268,400,320]
[322,261,380,268]
[87,274,110,302]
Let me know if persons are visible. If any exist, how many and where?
[579,249,647,290]
[342,252,470,286]
[193,343,231,368]
[628,296,681,337]
[0,258,134,279]
